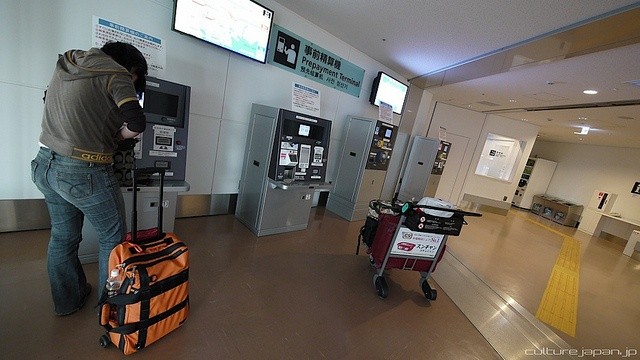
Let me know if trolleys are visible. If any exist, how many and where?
[361,199,482,300]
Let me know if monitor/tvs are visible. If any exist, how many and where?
[173,0,272,64]
[373,73,408,116]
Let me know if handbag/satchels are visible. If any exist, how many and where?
[110,138,141,187]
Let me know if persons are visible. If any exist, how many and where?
[31,41,147,314]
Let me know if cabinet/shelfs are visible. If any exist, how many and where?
[530,194,584,227]
[595,211,640,227]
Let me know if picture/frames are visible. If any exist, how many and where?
[631,181,640,195]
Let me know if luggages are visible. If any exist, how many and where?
[97,167,193,357]
